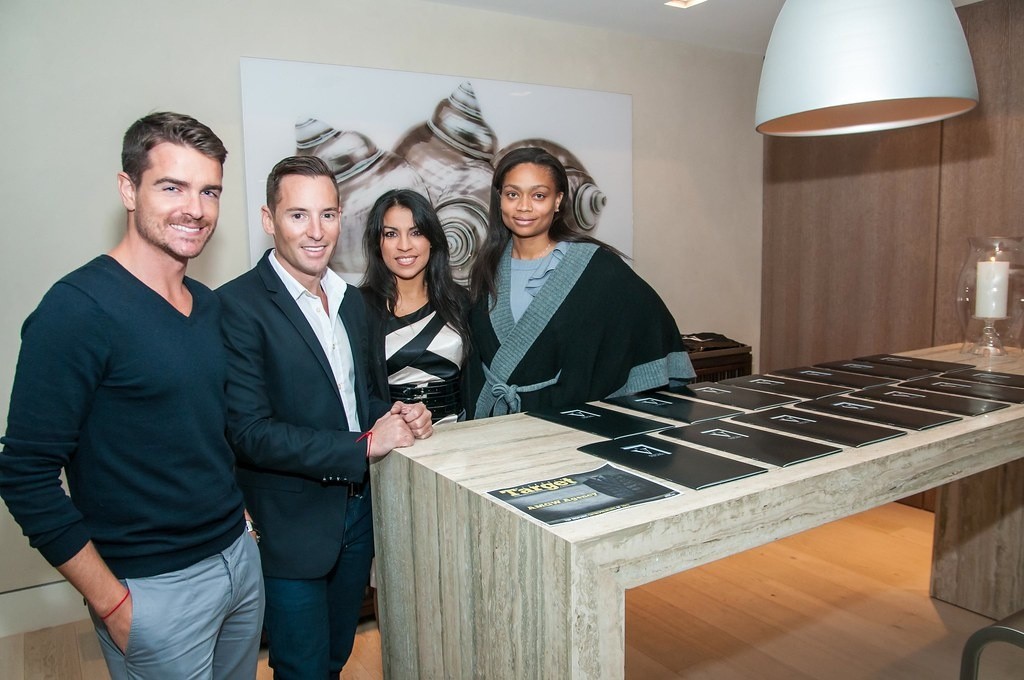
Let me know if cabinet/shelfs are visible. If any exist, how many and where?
[681,333,753,385]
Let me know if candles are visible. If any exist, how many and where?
[957,234,1024,360]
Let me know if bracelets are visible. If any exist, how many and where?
[99,588,130,622]
[355,431,373,459]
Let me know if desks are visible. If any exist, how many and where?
[364,341,1024,680]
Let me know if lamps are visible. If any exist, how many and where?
[755,2,979,138]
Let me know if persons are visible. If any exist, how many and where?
[468,147,696,421]
[353,189,472,426]
[2,111,267,680]
[214,156,435,680]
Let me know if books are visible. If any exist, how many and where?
[525,351,1024,492]
